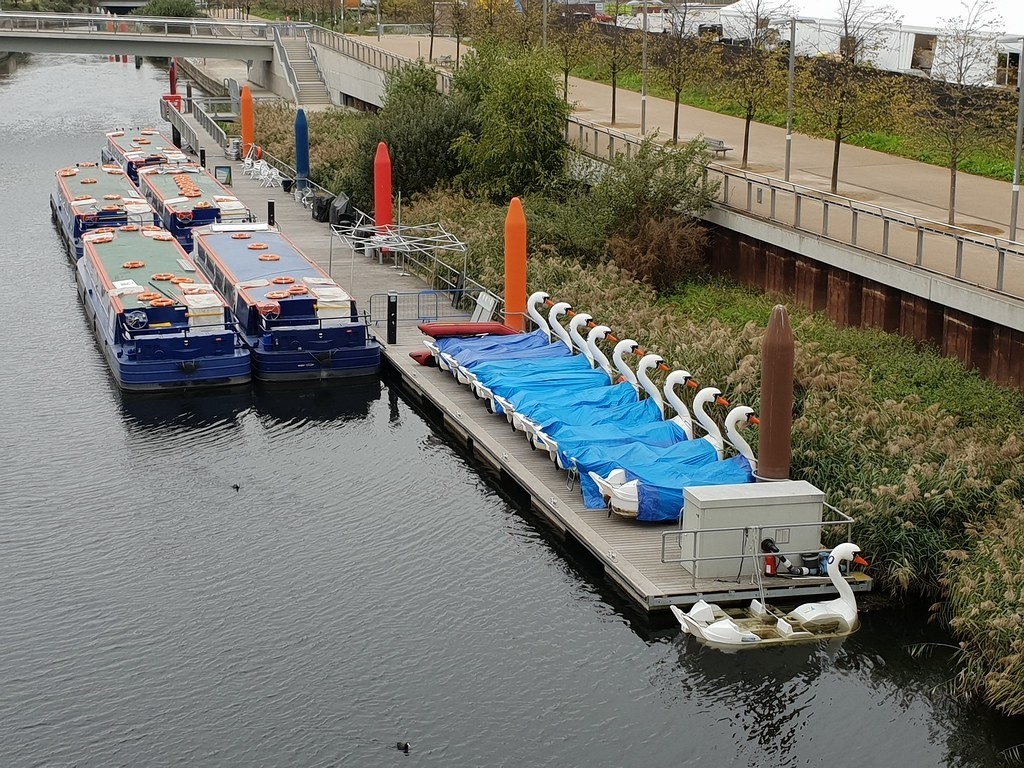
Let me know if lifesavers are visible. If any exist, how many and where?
[151,273,194,284]
[231,233,251,239]
[255,145,262,159]
[173,174,212,209]
[101,205,119,211]
[137,292,175,307]
[258,254,280,261]
[247,242,269,249]
[92,227,116,244]
[119,225,174,241]
[273,276,295,284]
[59,162,125,201]
[264,283,310,300]
[111,130,175,158]
[122,261,145,268]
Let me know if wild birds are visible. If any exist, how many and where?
[230,483,240,492]
[397,741,411,753]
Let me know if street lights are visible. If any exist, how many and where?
[628,1,663,134]
[997,36,1024,241]
[772,19,817,182]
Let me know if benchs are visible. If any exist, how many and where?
[702,136,733,158]
[434,55,451,65]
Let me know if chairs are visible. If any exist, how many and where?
[690,600,730,621]
[706,618,761,641]
[241,158,284,188]
[777,618,813,638]
[750,599,786,618]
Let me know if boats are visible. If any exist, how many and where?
[421,286,759,522]
[49,161,155,263]
[101,127,191,186]
[75,224,254,392]
[188,222,382,383]
[134,161,253,254]
[669,543,868,654]
